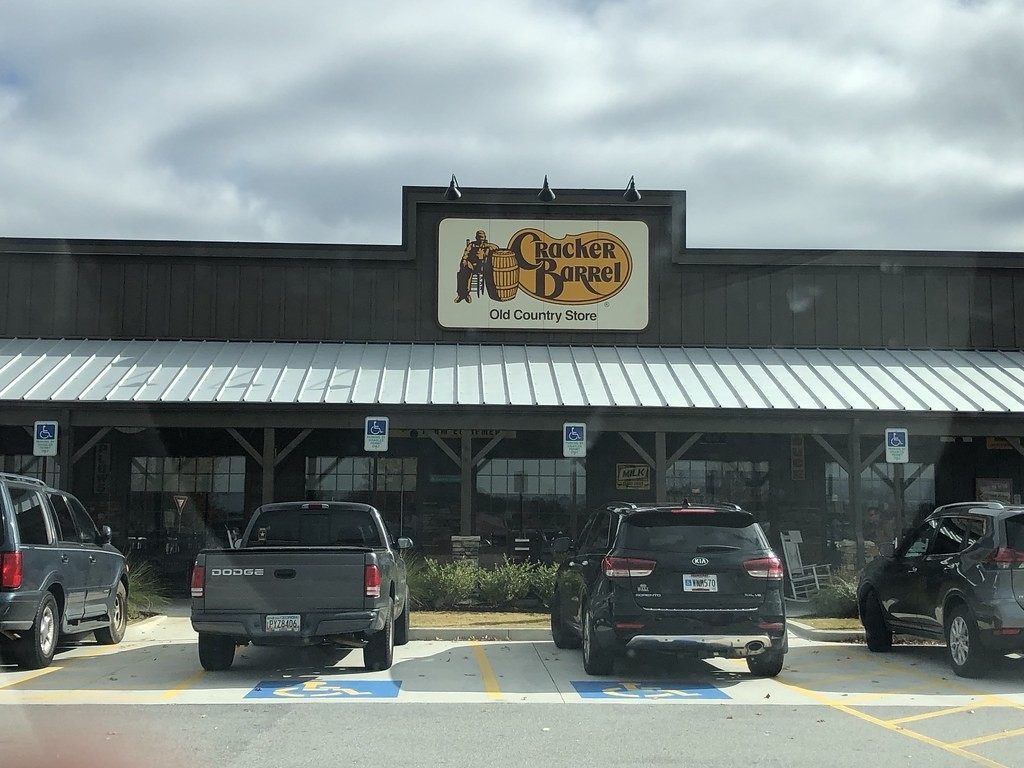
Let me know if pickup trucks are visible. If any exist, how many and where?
[191,501,415,673]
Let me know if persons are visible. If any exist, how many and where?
[835,499,897,553]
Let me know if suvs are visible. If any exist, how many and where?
[551,502,788,678]
[0,473,131,669]
[857,500,1023,678]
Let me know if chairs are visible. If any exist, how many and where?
[780,531,834,602]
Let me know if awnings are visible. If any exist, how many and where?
[2,344,1023,553]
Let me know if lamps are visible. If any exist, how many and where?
[537,175,556,201]
[623,176,642,202]
[443,173,462,200]
